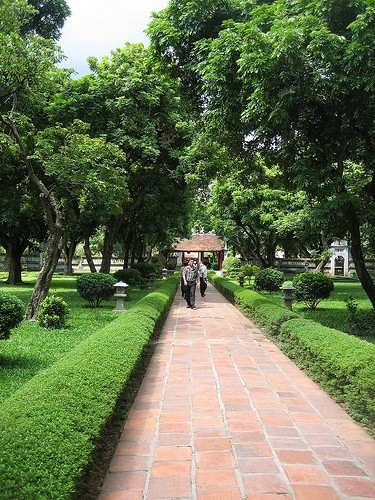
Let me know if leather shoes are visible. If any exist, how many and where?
[186,304,191,308]
[191,305,196,309]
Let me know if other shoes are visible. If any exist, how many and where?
[201,294,205,297]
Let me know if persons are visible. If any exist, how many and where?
[180,262,189,298]
[182,258,198,309]
[198,260,210,297]
[181,254,219,294]
